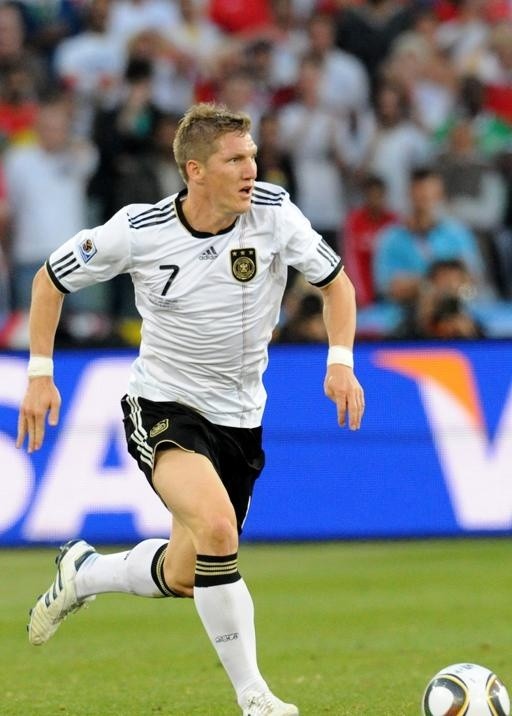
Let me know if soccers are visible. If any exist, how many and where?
[422,661,509,716]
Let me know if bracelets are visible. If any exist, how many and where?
[29,355,55,378]
[326,346,355,369]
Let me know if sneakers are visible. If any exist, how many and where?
[242,688,298,716]
[26,539,99,647]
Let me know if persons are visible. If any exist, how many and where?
[16,98,365,715]
